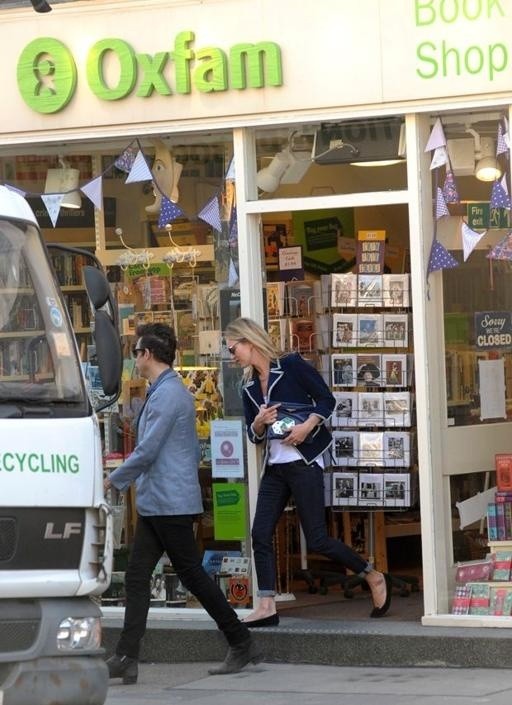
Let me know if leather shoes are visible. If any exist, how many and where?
[370,572,391,618]
[242,612,279,628]
[106,654,138,684]
[208,638,265,675]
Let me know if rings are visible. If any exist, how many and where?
[292,442,298,447]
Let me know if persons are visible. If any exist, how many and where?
[104,321,263,685]
[225,314,392,629]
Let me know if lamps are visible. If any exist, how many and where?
[474,136,503,183]
[256,151,290,194]
[153,147,184,204]
[42,146,82,209]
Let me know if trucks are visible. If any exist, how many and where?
[0,183,130,705]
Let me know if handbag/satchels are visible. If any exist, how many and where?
[266,401,315,445]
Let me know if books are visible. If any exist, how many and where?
[451,491,512,617]
[320,273,411,507]
[2,246,95,379]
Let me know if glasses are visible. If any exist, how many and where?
[228,341,243,355]
[131,349,145,358]
